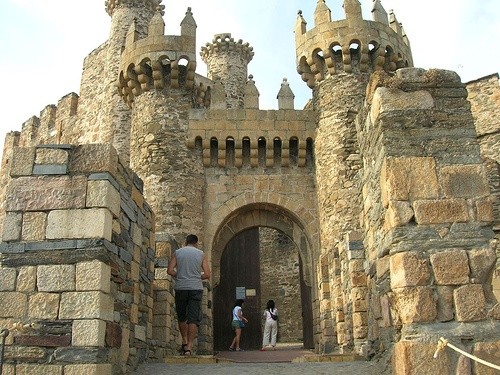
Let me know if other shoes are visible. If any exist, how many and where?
[271,347,277,351]
[236,348,244,353]
[181,343,188,350]
[261,348,266,351]
[183,350,192,356]
[228,346,236,351]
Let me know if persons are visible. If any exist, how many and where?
[167,234,210,355]
[228,299,248,352]
[260,300,278,351]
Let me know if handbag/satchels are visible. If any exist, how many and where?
[272,315,278,320]
[238,320,248,328]
